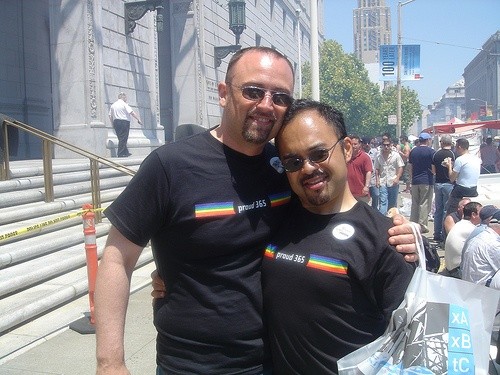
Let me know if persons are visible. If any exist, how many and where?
[109,92,143,157]
[149,97,417,375]
[92,47,423,375]
[346,132,500,364]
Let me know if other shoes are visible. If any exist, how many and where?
[434,234,444,240]
[428,214,434,222]
[401,182,406,185]
[399,190,410,194]
[421,225,429,232]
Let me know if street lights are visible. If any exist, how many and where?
[471,98,487,114]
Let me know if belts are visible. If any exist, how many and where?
[446,266,460,274]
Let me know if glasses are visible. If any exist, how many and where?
[226,81,295,107]
[281,136,348,173]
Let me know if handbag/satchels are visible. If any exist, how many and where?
[338,223,500,375]
[399,197,412,217]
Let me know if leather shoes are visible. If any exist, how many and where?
[118,153,132,157]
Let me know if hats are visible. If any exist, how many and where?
[479,205,500,220]
[362,137,370,144]
[419,133,431,139]
[490,211,500,224]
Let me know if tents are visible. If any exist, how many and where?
[420,117,500,152]
